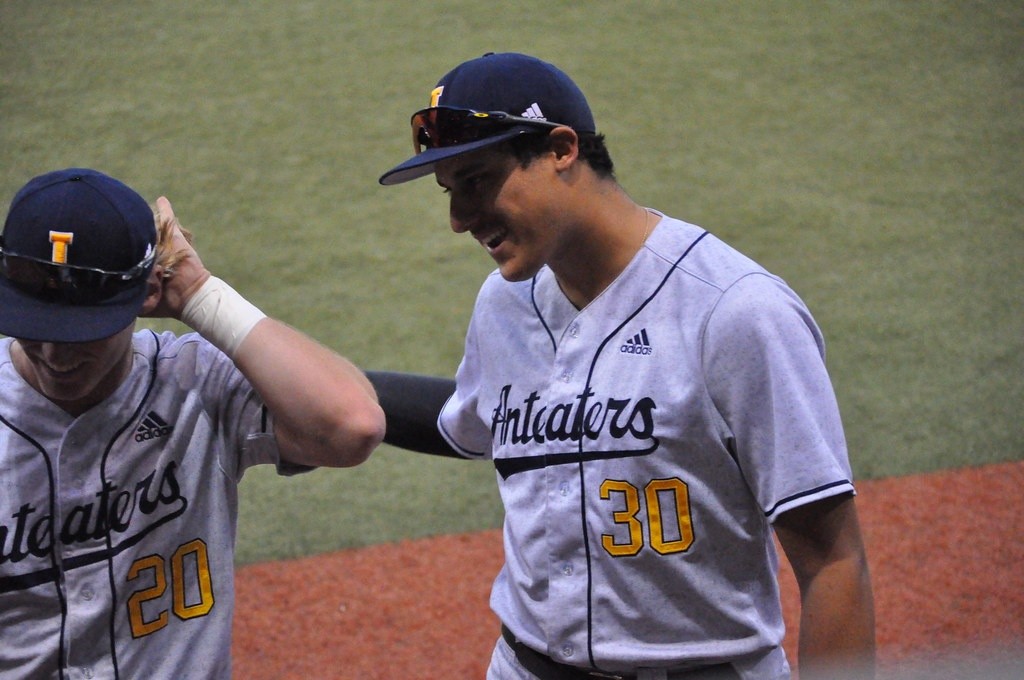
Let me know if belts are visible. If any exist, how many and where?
[502,623,732,680]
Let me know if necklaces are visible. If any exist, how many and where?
[639,206,651,248]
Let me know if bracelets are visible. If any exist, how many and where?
[181,274,268,359]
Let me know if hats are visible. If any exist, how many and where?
[0,169,158,342]
[378,53,596,185]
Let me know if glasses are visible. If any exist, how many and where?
[0,249,155,305]
[410,107,572,155]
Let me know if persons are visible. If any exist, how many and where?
[359,51,877,680]
[2,166,388,680]
